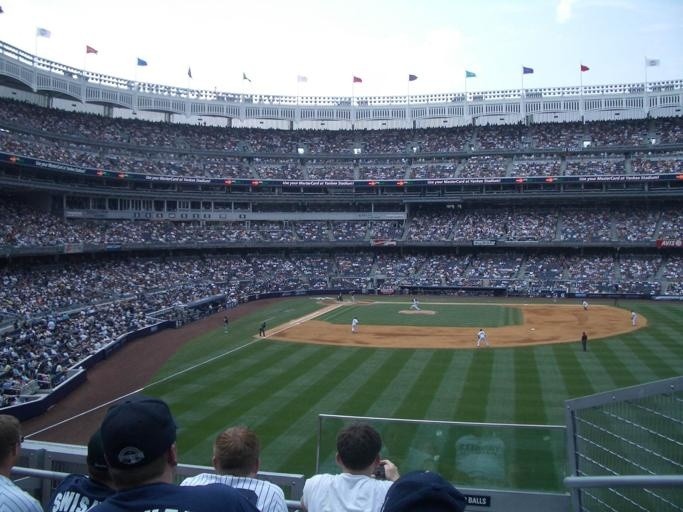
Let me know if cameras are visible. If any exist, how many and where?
[373,463,385,477]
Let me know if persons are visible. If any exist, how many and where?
[248,125,683,297]
[408,297,422,312]
[351,293,355,302]
[0,413,45,512]
[582,300,589,310]
[0,94,250,409]
[83,397,261,512]
[630,310,638,328]
[581,332,588,353]
[41,428,120,512]
[179,424,290,512]
[351,316,359,334]
[259,321,267,337]
[476,327,489,348]
[300,421,402,512]
[338,292,343,302]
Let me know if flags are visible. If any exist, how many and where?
[187,67,192,78]
[35,27,51,38]
[138,57,148,67]
[522,66,534,74]
[645,58,660,68]
[86,45,98,55]
[408,74,418,81]
[580,64,590,73]
[465,70,476,78]
[243,72,249,81]
[0,6,4,14]
[352,75,362,83]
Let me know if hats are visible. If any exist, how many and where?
[86,429,112,472]
[98,395,178,469]
